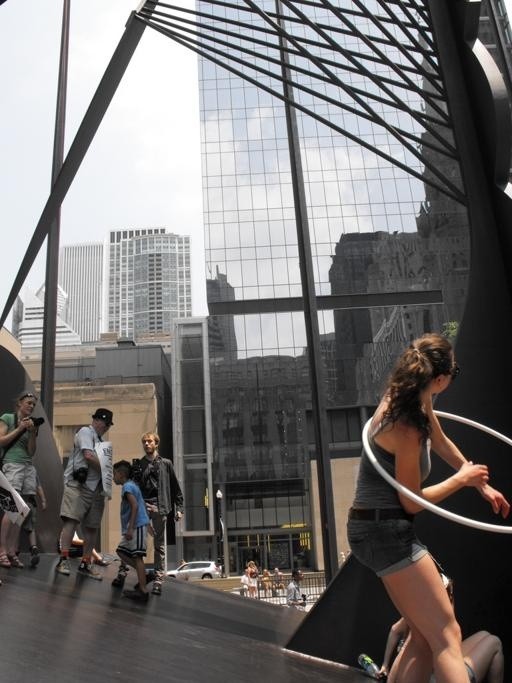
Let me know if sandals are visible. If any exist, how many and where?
[0,552,11,567]
[10,552,24,568]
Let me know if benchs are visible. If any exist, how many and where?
[232,584,288,596]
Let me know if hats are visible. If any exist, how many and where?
[90,407,115,425]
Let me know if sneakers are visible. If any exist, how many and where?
[151,581,162,595]
[111,571,126,587]
[79,559,105,581]
[29,545,41,566]
[54,558,72,576]
[124,587,150,604]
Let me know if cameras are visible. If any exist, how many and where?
[28,416,45,427]
[72,467,87,483]
[131,459,142,482]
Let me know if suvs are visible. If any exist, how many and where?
[166,559,223,581]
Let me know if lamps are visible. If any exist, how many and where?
[214,486,223,499]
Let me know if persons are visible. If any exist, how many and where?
[18,475,48,568]
[237,560,308,609]
[58,523,113,567]
[112,459,150,600]
[56,408,114,581]
[0,390,39,568]
[346,335,512,683]
[114,430,184,594]
[375,570,505,683]
